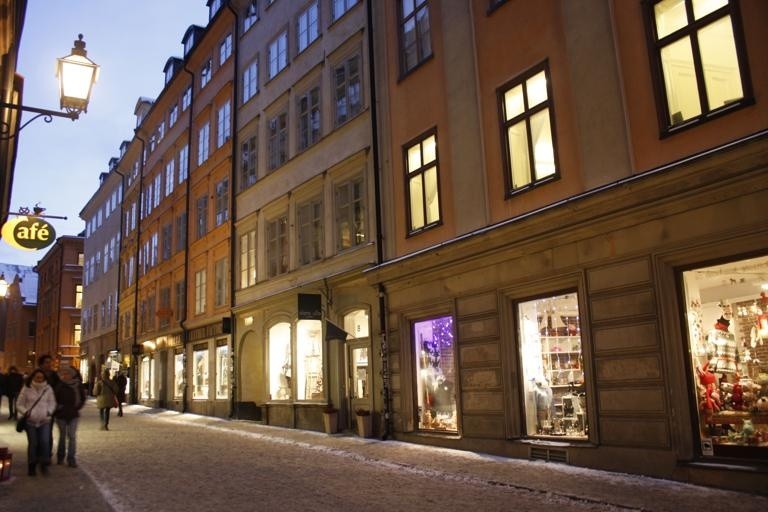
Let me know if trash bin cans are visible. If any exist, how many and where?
[356,410,370,438]
[323,409,338,434]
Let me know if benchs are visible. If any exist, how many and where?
[705,409,768,425]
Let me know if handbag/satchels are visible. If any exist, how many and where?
[112,395,118,408]
[16,413,29,432]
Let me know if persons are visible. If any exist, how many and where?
[112,371,117,380]
[114,370,127,417]
[706,316,743,374]
[36,354,70,467]
[54,364,86,469]
[6,366,24,420]
[23,371,29,378]
[0,366,5,408]
[15,368,58,477]
[92,371,118,430]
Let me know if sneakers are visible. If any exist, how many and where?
[27,469,36,476]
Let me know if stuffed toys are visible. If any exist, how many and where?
[694,361,768,447]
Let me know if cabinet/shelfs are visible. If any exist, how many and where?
[559,396,587,437]
[539,312,584,416]
